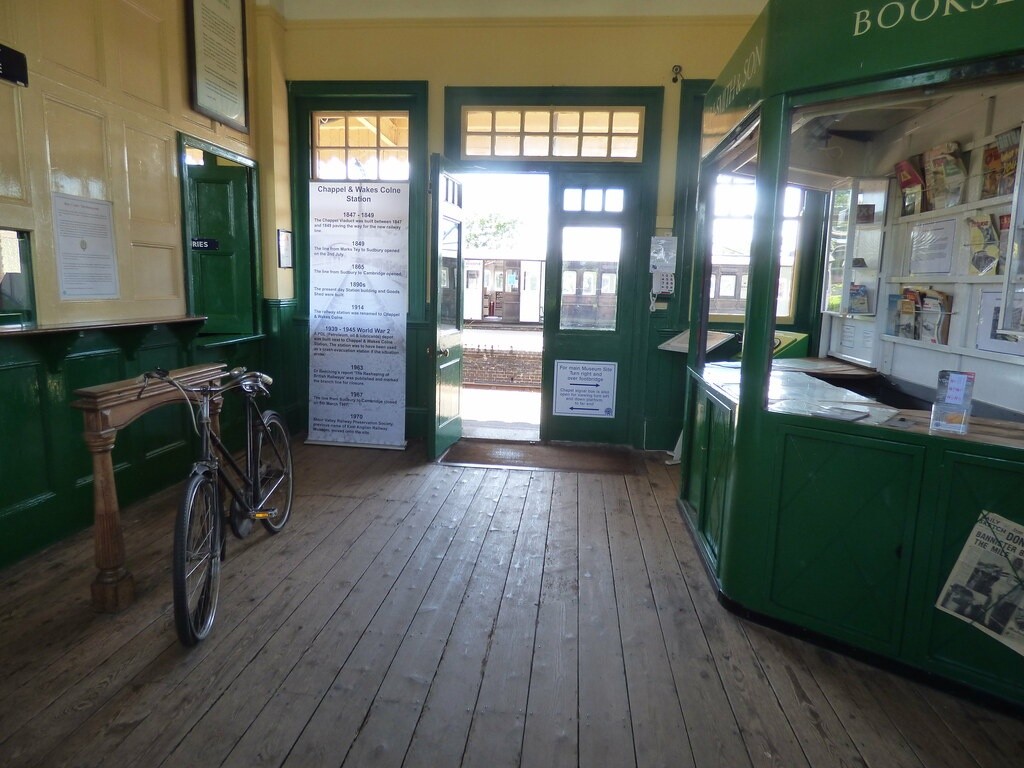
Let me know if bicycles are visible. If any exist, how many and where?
[136,364,294,647]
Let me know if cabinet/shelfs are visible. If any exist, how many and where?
[916,436,1023,710]
[758,410,928,658]
[698,383,739,578]
[681,365,706,533]
